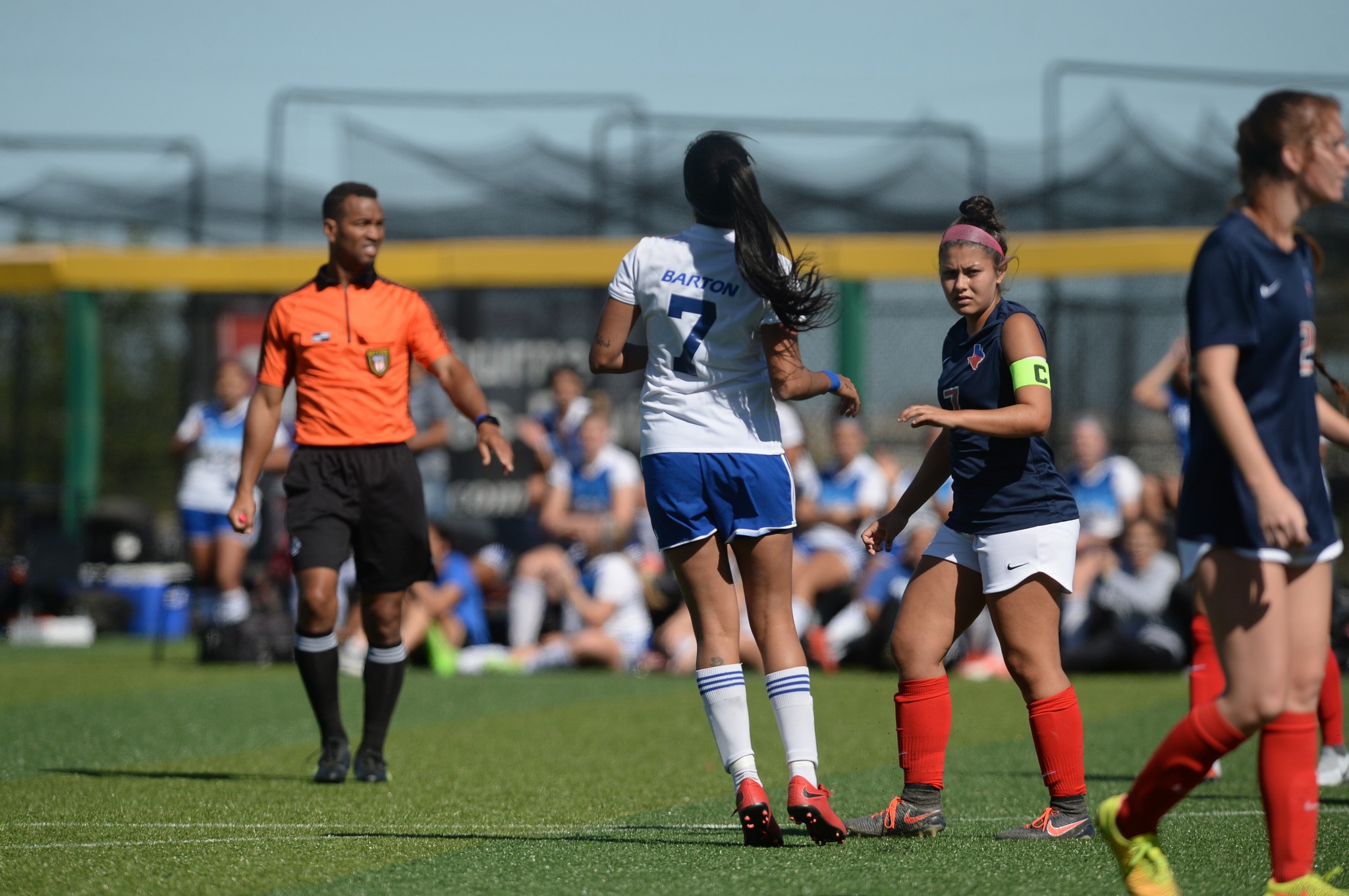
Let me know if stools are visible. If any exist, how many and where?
[154,574,279,651]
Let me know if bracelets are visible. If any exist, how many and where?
[819,369,841,394]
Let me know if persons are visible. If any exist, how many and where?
[585,126,863,846]
[223,179,519,782]
[172,355,291,665]
[1091,82,1349,896]
[288,364,1349,683]
[1133,334,1348,791]
[843,193,1099,842]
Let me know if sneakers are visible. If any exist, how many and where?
[844,796,946,837]
[787,775,846,846]
[1096,794,1181,896]
[1262,867,1348,896]
[996,809,1095,840]
[730,778,784,847]
[352,744,393,781]
[306,737,351,783]
[1315,746,1349,787]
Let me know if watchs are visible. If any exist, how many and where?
[473,415,500,431]
